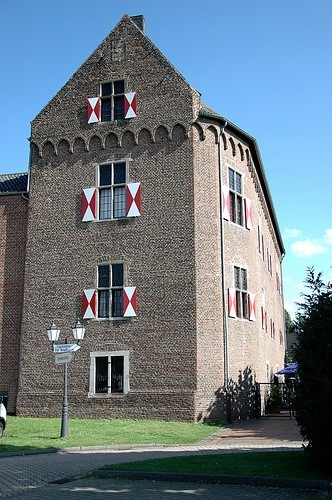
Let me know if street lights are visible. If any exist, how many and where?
[44,317,85,437]
